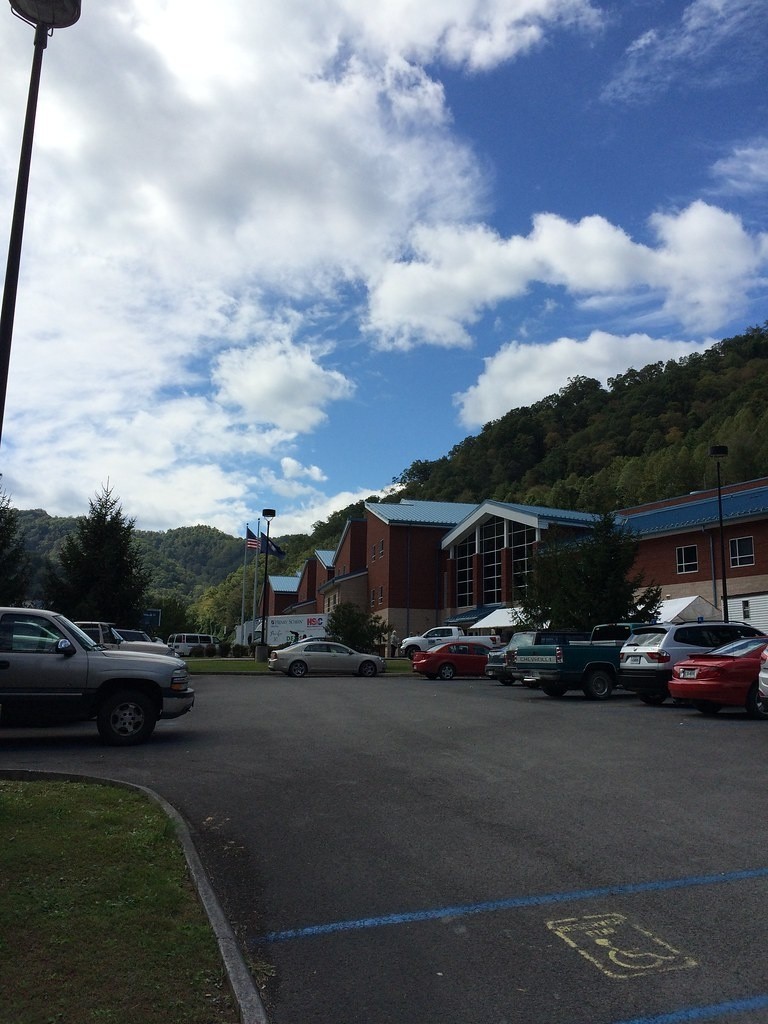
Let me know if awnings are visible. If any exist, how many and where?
[442,605,504,624]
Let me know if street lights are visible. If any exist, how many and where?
[255,508,276,664]
[0,1,83,489]
[709,446,732,624]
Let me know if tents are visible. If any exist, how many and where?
[469,596,723,643]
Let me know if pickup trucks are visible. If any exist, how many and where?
[400,626,502,661]
[512,621,664,701]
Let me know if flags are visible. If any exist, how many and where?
[247,528,261,549]
[260,532,286,560]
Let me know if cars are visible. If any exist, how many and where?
[412,642,492,680]
[668,636,768,718]
[71,621,179,660]
[758,643,768,712]
[114,628,154,644]
[294,636,345,649]
[268,641,387,678]
[8,621,70,654]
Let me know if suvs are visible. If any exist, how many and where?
[166,634,179,650]
[619,620,767,708]
[0,605,199,746]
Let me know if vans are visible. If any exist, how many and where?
[173,634,228,658]
[485,630,604,687]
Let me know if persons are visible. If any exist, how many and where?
[390,629,400,658]
[408,632,414,637]
[415,632,420,636]
